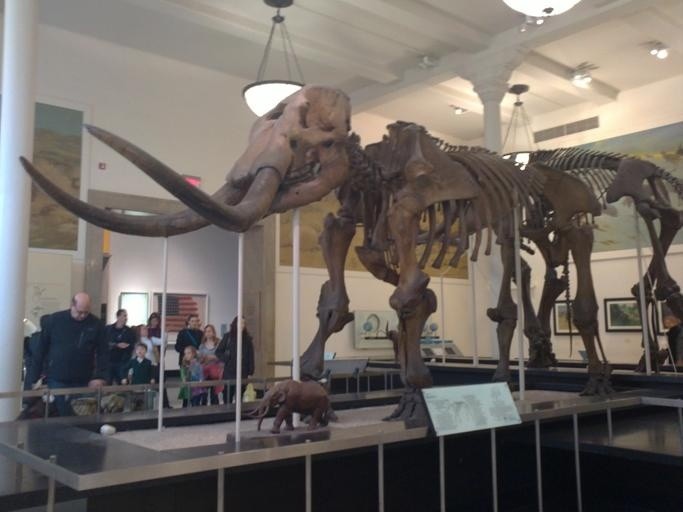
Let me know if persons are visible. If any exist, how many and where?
[22,291,255,418]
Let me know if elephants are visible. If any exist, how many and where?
[241,379,330,433]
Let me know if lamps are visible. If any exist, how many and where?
[504,0,589,22]
[241,0,309,118]
[499,83,544,172]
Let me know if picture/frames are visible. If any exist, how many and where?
[655,297,675,336]
[23,94,92,257]
[604,296,645,334]
[149,289,210,347]
[119,291,149,328]
[552,299,586,337]
